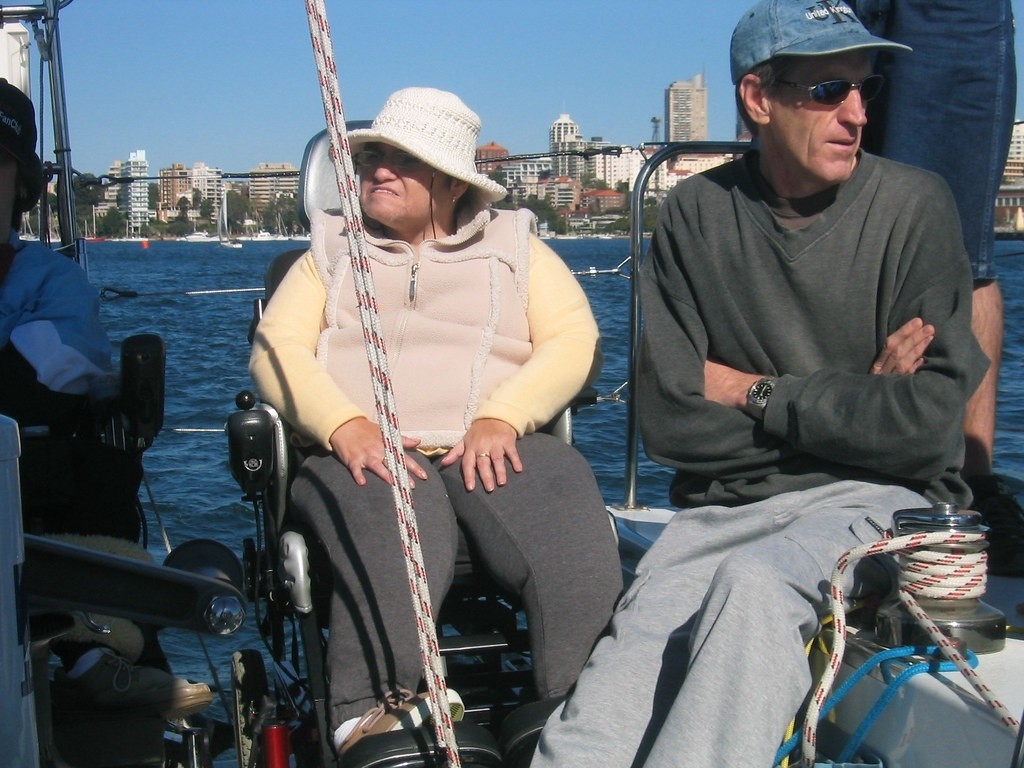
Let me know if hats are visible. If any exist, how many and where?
[730,0,913,85]
[0,78,43,212]
[329,87,508,205]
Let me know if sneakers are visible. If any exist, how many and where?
[337,687,465,762]
[54,647,212,721]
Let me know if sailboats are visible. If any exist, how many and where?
[220,190,244,249]
[19,198,105,242]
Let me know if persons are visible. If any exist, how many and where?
[531,0,992,768]
[248,84,625,762]
[0,75,216,715]
[838,0,1024,575]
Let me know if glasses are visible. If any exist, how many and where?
[354,149,428,168]
[778,75,885,106]
[0,148,15,165]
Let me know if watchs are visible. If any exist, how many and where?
[744,375,776,419]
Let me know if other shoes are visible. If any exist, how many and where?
[965,474,1024,576]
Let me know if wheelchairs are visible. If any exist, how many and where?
[225,245,601,768]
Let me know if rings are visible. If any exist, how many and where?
[476,452,489,457]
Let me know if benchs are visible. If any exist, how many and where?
[600,506,1024,768]
